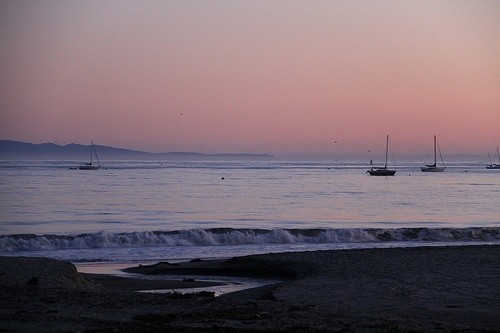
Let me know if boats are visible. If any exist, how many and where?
[420,135,446,172]
[79,140,101,169]
[486,147,500,169]
[365,134,397,176]
